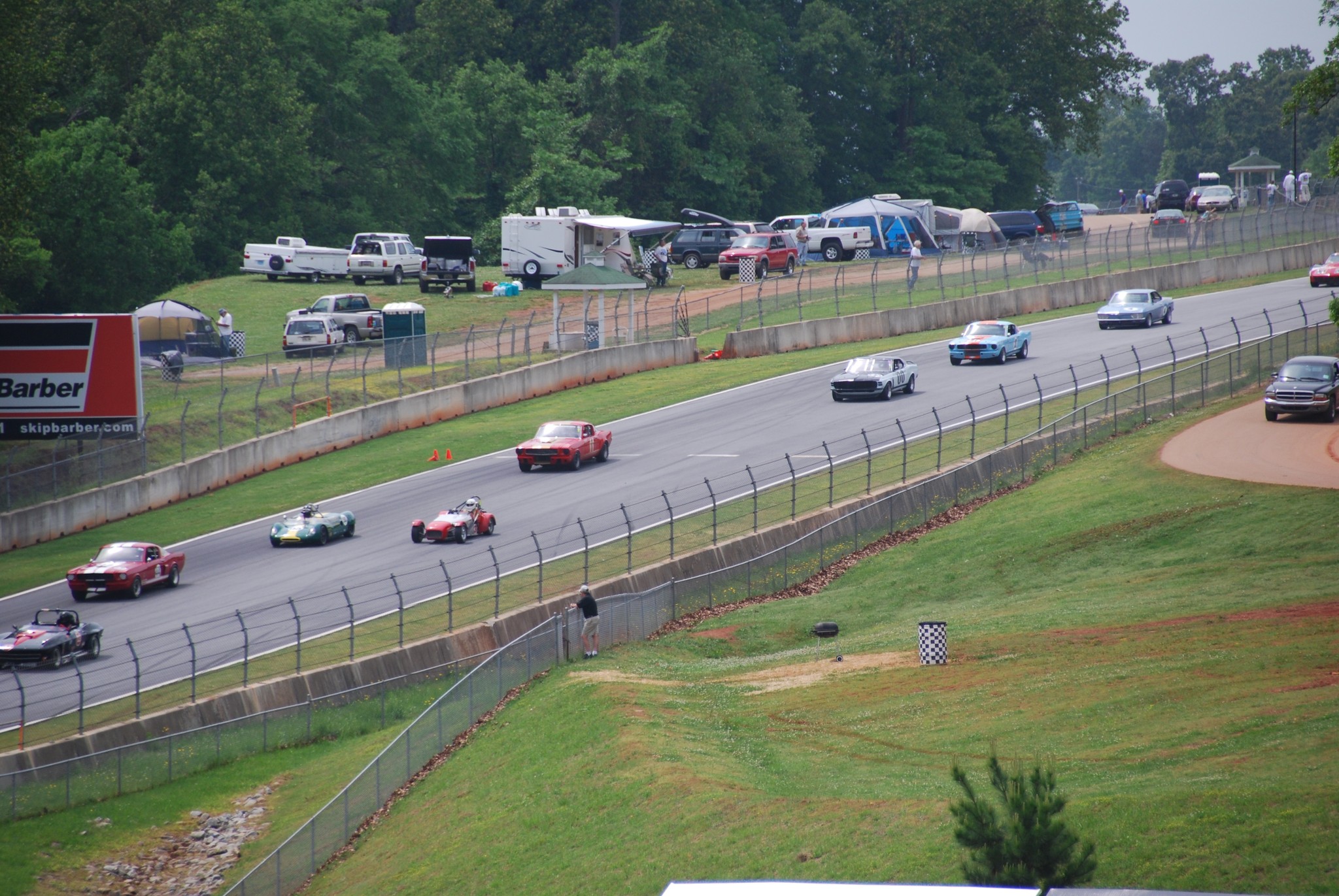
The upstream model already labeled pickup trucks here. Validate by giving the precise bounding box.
[768,215,874,263]
[285,293,383,347]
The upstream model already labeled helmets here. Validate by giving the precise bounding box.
[466,499,476,512]
[59,611,75,627]
[303,505,312,516]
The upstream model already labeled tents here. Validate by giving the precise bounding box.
[132,299,226,357]
[818,195,1009,260]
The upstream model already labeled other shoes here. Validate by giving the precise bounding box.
[582,653,597,659]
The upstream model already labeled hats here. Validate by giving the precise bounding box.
[580,585,589,592]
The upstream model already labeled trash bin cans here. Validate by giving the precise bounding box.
[229,331,245,356]
[918,621,948,664]
[962,232,977,254]
[855,249,869,260]
[161,353,181,381]
[584,321,599,350]
[382,301,428,370]
[739,257,755,283]
[644,248,657,272]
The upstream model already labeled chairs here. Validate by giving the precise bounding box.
[620,259,657,286]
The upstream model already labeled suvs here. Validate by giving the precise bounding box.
[414,235,481,293]
[352,232,424,256]
[718,229,798,280]
[282,314,345,359]
[707,222,775,234]
[346,239,424,286]
[1150,179,1190,215]
[671,207,747,269]
[986,209,1045,252]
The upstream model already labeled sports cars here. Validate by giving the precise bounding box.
[1310,253,1339,287]
[1097,289,1174,330]
[830,356,919,402]
[411,495,497,544]
[66,542,185,601]
[0,609,104,671]
[270,503,356,547]
[515,421,612,472]
[948,320,1031,366]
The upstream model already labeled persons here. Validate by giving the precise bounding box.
[1135,189,1157,214]
[216,308,232,358]
[1021,244,1055,271]
[570,585,599,660]
[906,240,926,293]
[300,505,312,519]
[795,221,809,267]
[654,238,668,288]
[1257,184,1262,206]
[1267,179,1279,210]
[1190,204,1223,250]
[1282,170,1298,205]
[1298,169,1311,204]
[55,611,75,631]
[1118,189,1128,215]
[462,498,481,523]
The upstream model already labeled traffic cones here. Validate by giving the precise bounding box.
[433,449,440,461]
[428,455,437,462]
[446,449,453,460]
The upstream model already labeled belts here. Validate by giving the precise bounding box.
[587,615,598,618]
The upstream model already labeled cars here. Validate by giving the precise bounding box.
[1034,202,1084,239]
[1184,185,1207,212]
[1069,203,1104,216]
[1264,355,1339,423]
[1150,209,1188,238]
[1198,185,1238,214]
